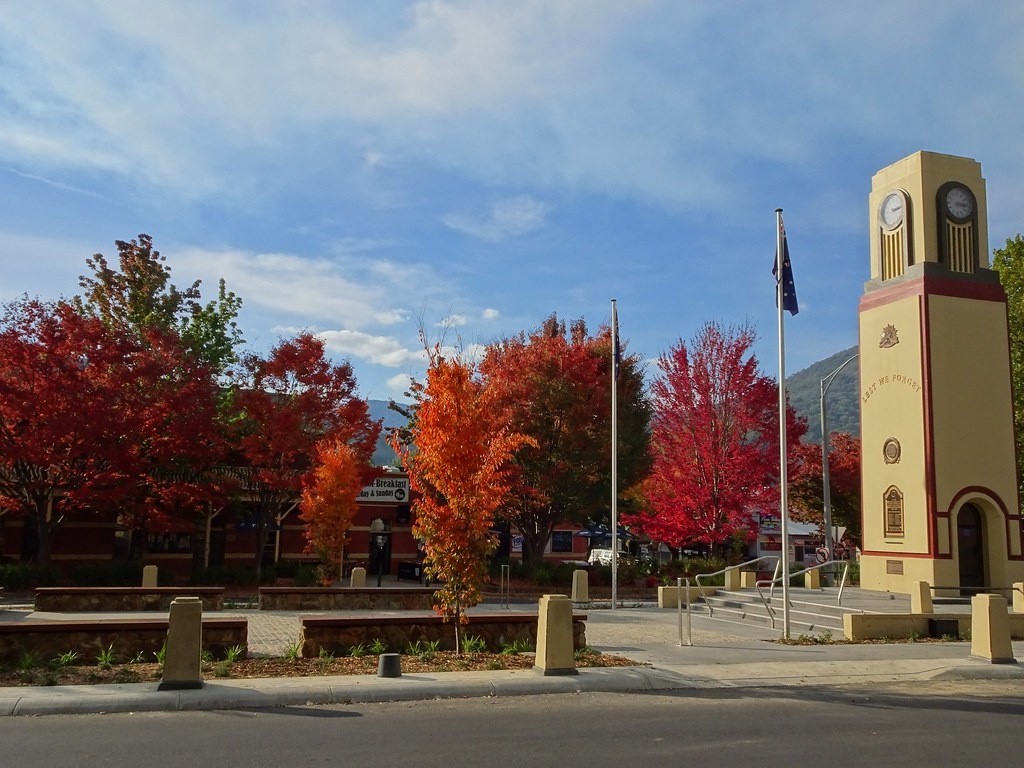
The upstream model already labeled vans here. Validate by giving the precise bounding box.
[589,548,639,566]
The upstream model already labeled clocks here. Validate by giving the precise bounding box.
[877,192,903,231]
[943,183,976,224]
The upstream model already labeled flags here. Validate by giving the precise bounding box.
[612,299,620,383]
[772,213,799,316]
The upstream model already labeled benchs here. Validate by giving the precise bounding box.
[34,587,225,611]
[0,617,248,664]
[299,609,587,658]
[258,587,443,611]
